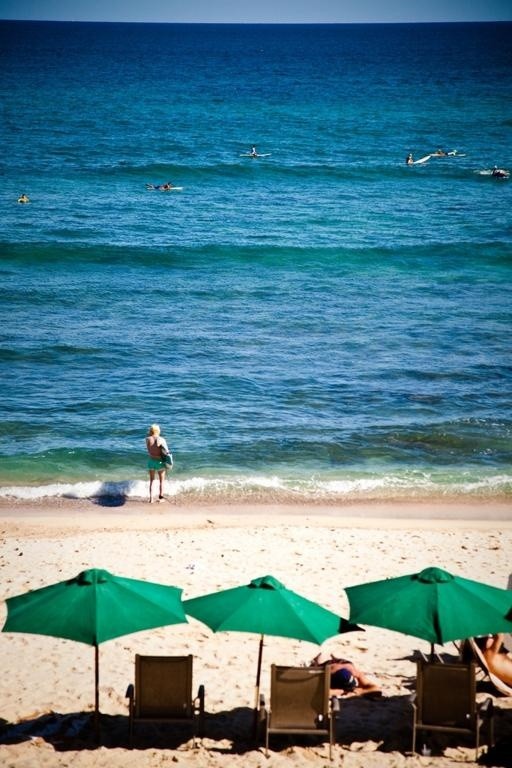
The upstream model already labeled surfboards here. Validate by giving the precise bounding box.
[412,155,432,162]
[239,153,271,158]
[146,186,184,190]
[161,448,173,470]
[429,152,465,158]
[475,169,510,175]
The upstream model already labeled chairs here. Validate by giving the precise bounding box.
[264,663,335,761]
[124,653,206,743]
[411,650,496,762]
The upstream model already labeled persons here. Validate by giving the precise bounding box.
[310,656,379,701]
[473,632,512,689]
[145,183,170,190]
[492,165,508,177]
[145,424,169,504]
[429,149,458,157]
[17,194,30,203]
[251,145,256,157]
[407,153,413,165]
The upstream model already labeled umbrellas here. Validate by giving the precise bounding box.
[181,575,366,713]
[344,567,512,666]
[1,568,188,714]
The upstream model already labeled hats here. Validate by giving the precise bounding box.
[334,668,357,688]
